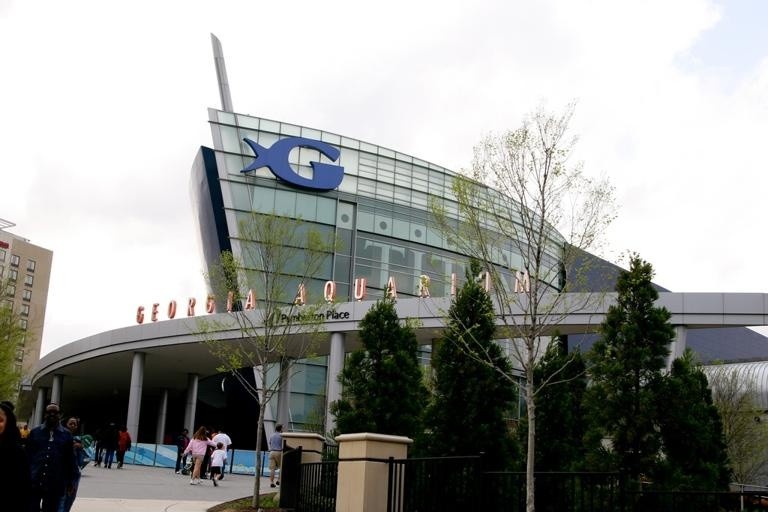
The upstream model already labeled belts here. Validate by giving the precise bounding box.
[270,450,282,451]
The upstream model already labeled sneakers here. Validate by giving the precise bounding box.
[98,463,100,465]
[108,466,111,469]
[276,481,280,485]
[94,463,97,466]
[176,470,224,486]
[271,484,276,488]
[120,465,123,467]
[104,465,107,468]
[117,464,120,468]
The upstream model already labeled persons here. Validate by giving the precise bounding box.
[269,423,285,488]
[1,399,233,512]
